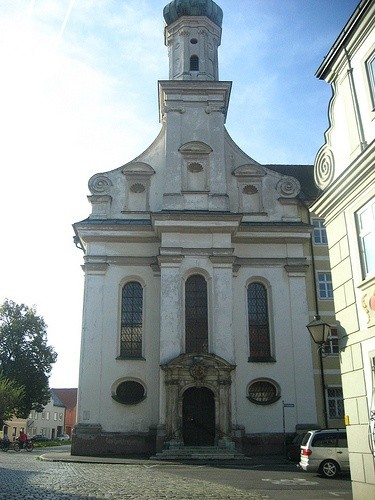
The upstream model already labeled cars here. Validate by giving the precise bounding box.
[29,435,49,442]
[56,434,70,441]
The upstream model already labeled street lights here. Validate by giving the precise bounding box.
[306,314,331,429]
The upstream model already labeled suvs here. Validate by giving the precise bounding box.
[297,427,350,480]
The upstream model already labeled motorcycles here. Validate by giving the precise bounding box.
[11,439,34,452]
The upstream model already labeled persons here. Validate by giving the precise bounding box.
[18,432,27,450]
